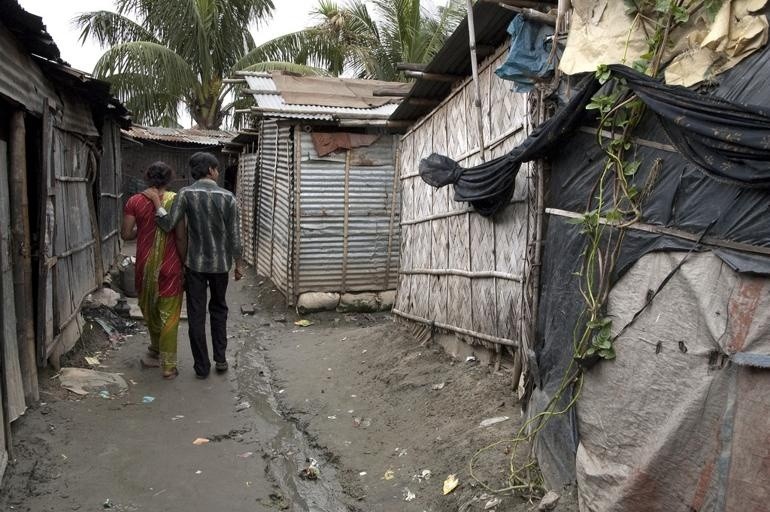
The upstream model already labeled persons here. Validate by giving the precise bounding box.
[121,151,244,380]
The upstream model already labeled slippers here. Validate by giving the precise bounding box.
[147,343,159,358]
[164,367,178,379]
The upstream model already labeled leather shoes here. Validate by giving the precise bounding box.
[194,364,211,379]
[213,360,229,374]
